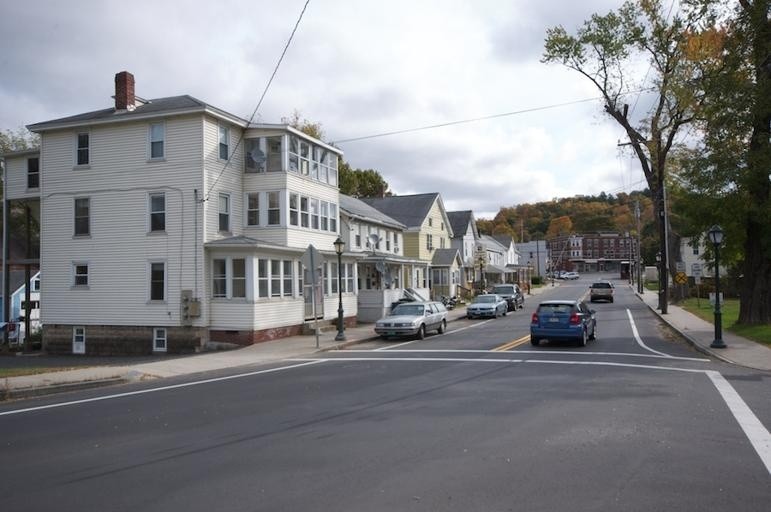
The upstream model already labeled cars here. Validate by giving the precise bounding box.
[375,301,448,339]
[589,280,614,302]
[467,294,507,320]
[546,270,578,280]
[530,300,596,347]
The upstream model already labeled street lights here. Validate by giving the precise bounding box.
[334,238,346,340]
[709,224,727,347]
[656,251,662,309]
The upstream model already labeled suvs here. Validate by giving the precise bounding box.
[488,284,524,311]
